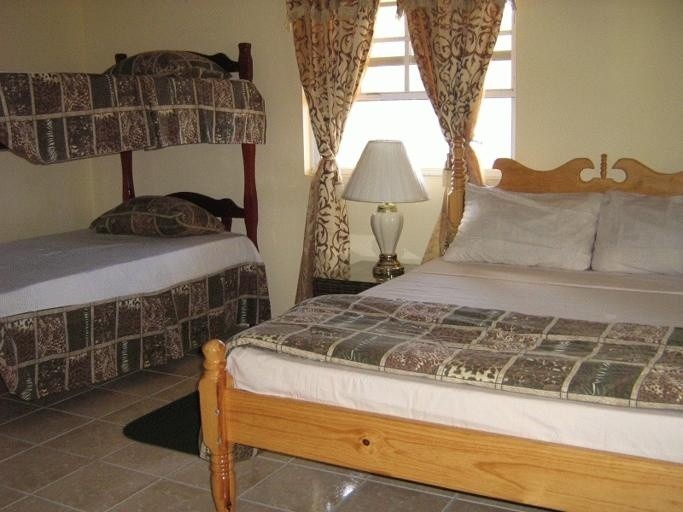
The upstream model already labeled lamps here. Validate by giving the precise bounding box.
[341,140,429,278]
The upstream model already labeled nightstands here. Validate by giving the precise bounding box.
[314,261,420,294]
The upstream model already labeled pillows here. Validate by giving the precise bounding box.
[445,185,602,271]
[89,195,226,236]
[104,50,232,77]
[593,189,683,274]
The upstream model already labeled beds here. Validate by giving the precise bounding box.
[201,153,683,511]
[0,44,273,404]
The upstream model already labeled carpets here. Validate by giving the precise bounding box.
[122,391,200,456]
